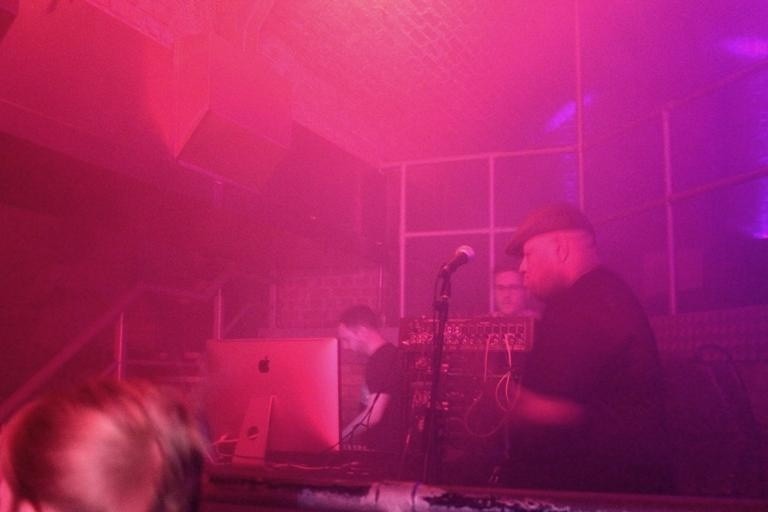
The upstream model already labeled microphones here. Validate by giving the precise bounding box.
[435,244,478,280]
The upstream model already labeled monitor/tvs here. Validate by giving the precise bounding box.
[200,336,347,469]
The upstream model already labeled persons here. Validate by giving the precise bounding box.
[1,377,204,511]
[491,258,528,318]
[338,305,409,469]
[483,201,662,490]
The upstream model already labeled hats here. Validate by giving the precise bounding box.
[502,204,595,259]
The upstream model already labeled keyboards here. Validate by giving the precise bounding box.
[211,466,374,496]
